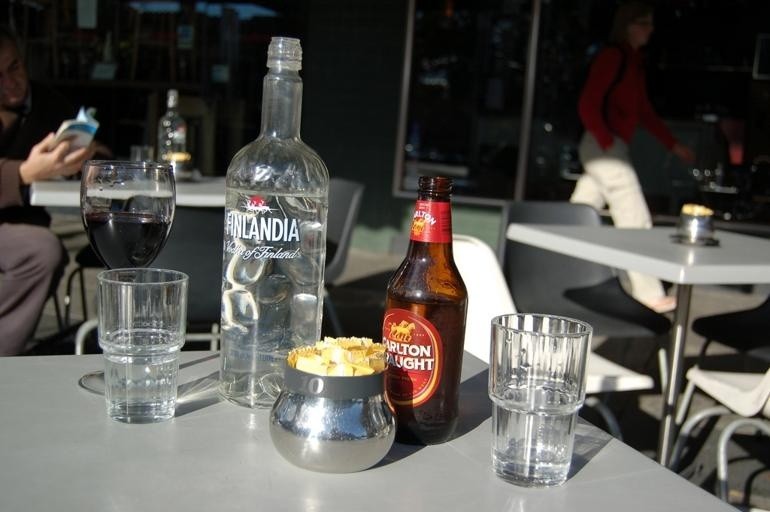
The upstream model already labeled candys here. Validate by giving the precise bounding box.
[294,336,388,376]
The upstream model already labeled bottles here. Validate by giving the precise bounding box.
[157,90,187,166]
[382,176,468,445]
[221,35,330,412]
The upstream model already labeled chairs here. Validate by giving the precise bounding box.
[504,200,671,367]
[142,204,224,322]
[674,365,770,501]
[322,177,365,336]
[452,232,655,443]
[65,243,106,328]
[692,290,770,365]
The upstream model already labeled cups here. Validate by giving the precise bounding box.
[96,267,190,423]
[487,313,592,488]
[131,145,153,181]
[79,159,176,398]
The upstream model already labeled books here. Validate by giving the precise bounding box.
[47,105,101,150]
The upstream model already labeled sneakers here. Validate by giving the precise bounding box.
[647,296,677,315]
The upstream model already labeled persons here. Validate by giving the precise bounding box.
[0,131,95,357]
[0,34,44,131]
[569,9,695,313]
